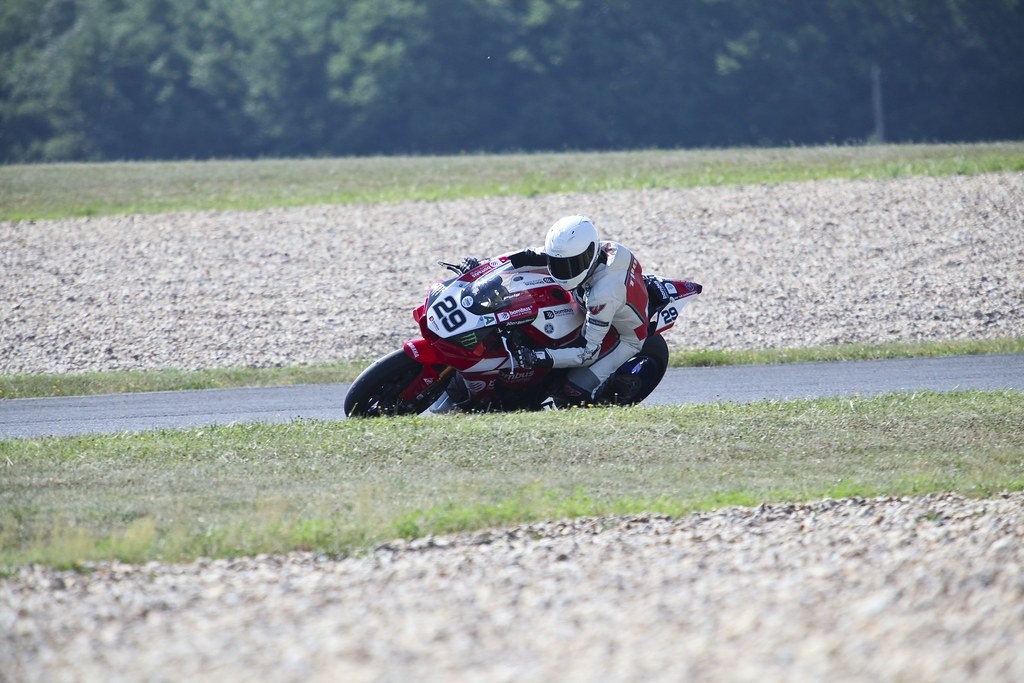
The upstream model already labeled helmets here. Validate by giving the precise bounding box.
[545,216,598,291]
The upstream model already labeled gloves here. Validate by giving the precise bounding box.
[460,256,481,273]
[514,346,553,370]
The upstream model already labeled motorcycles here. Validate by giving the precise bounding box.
[342,251,703,417]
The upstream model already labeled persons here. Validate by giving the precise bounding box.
[459,215,649,412]
